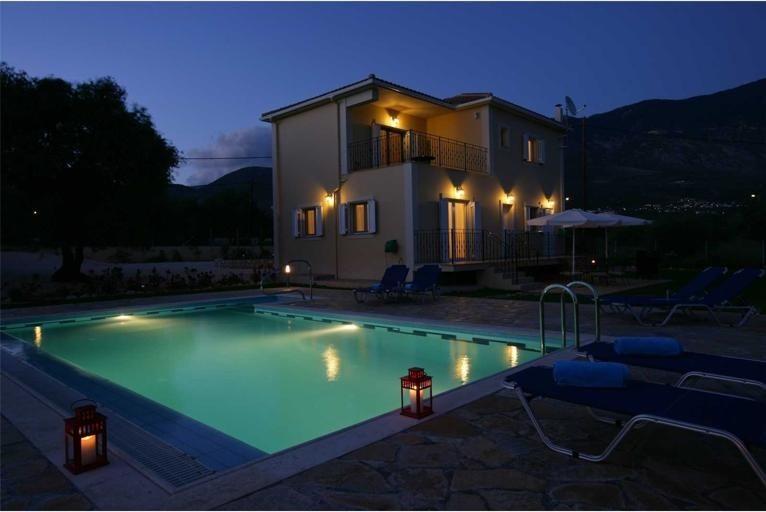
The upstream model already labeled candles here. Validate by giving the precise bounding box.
[80,433,96,464]
[410,386,424,412]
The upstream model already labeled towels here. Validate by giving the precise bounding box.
[613,336,681,357]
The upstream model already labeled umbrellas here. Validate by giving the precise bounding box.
[527,208,621,273]
[599,212,654,259]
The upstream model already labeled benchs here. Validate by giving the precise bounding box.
[500,340,764,489]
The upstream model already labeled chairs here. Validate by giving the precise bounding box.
[588,263,764,327]
[353,264,443,307]
